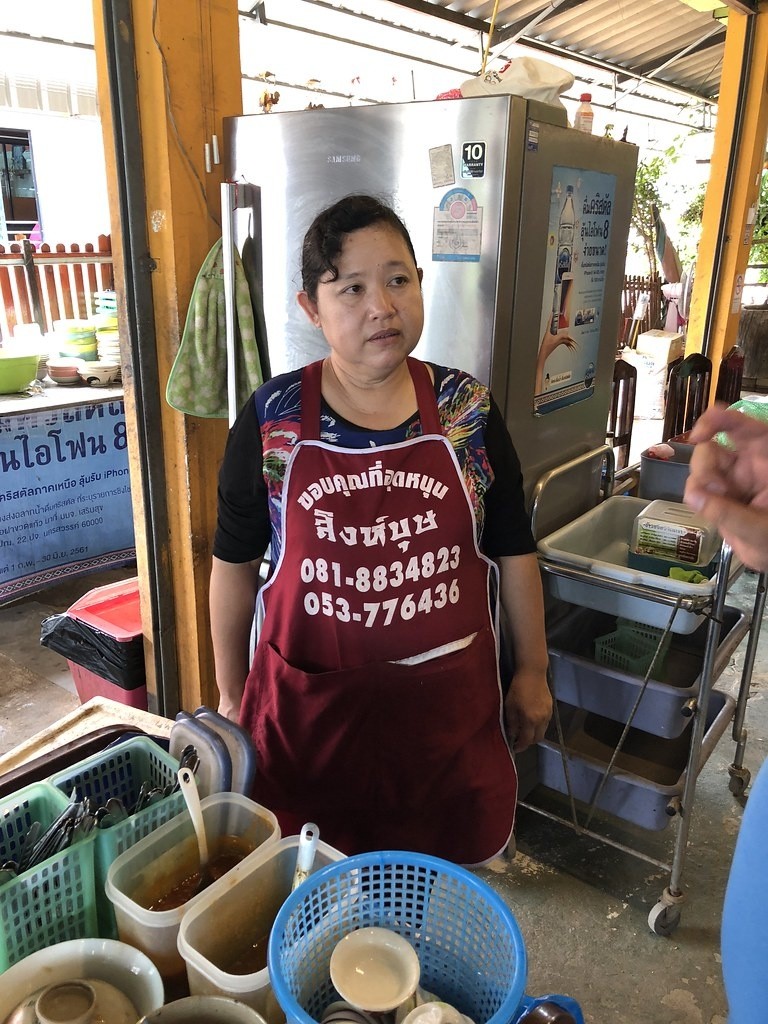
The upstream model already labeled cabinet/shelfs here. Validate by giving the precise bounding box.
[508,444,768,936]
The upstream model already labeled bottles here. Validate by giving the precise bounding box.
[574,94,593,133]
[553,185,576,312]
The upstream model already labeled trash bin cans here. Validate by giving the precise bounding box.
[60,576,149,712]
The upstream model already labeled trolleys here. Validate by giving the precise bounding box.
[501,442,768,937]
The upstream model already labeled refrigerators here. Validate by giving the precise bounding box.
[222,97,637,570]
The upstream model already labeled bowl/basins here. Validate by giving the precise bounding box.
[137,995,267,1024]
[7,978,137,1024]
[0,938,164,1024]
[0,319,119,394]
[322,927,475,1024]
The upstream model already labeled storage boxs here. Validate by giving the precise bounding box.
[547,605,751,740]
[537,496,746,635]
[637,442,695,503]
[538,689,736,833]
[636,329,684,364]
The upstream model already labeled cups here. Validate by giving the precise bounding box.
[558,271,574,328]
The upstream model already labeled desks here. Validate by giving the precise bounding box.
[0,376,136,606]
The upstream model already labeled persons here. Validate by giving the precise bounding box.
[681,406,768,1024]
[202,193,556,927]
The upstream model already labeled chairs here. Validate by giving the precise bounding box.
[714,344,745,406]
[598,359,637,506]
[661,352,713,443]
